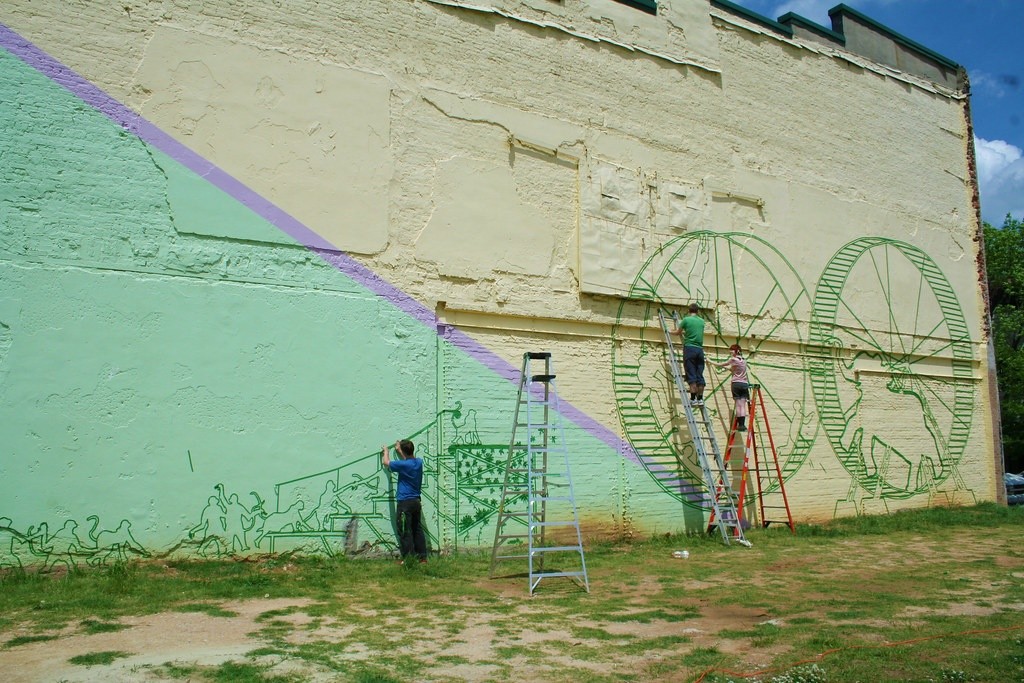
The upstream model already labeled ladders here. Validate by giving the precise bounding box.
[703,385,794,536]
[490,351,589,596]
[656,306,745,546]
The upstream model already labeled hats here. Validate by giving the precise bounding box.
[730,344,742,352]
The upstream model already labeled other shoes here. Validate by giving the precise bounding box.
[690,398,698,406]
[394,560,404,564]
[696,400,704,406]
[417,559,427,564]
[737,426,747,431]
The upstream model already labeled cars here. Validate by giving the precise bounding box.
[1005,470,1024,505]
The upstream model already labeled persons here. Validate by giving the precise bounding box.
[381,439,427,566]
[705,344,750,432]
[667,303,705,406]
